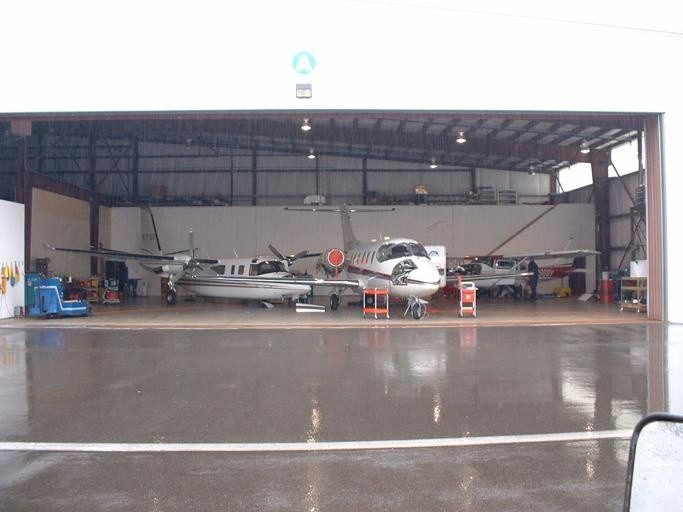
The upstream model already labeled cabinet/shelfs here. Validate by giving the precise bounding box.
[620,277,648,309]
[363,288,389,320]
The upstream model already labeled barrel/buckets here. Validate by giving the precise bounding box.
[600,280,614,302]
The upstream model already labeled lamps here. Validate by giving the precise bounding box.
[308,149,315,159]
[580,138,590,154]
[430,158,437,169]
[302,118,311,131]
[528,165,535,176]
[456,130,466,144]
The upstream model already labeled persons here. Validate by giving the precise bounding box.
[527,257,539,300]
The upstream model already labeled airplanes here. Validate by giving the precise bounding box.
[44,212,326,307]
[268,201,534,320]
